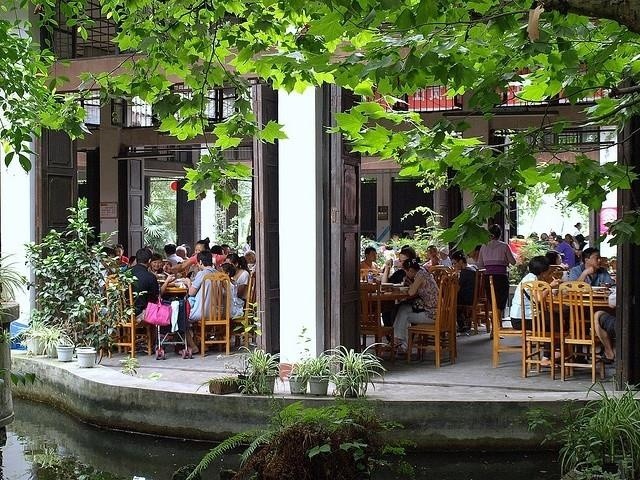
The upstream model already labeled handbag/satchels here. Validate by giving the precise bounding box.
[144,301,172,326]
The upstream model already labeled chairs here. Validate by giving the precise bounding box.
[359,264,619,384]
[86,250,256,361]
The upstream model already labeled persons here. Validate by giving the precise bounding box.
[361,223,617,369]
[93,237,256,354]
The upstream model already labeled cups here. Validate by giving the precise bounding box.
[551,282,616,300]
[157,270,194,287]
[401,282,407,287]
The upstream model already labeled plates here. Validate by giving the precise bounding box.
[393,284,402,287]
[382,283,394,286]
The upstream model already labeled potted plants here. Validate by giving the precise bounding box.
[18,312,98,368]
[561,382,640,480]
[195,342,390,396]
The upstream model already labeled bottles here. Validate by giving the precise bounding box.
[368,271,381,285]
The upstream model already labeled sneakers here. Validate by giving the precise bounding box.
[175,346,208,355]
[599,350,615,364]
[540,349,561,368]
[490,334,504,338]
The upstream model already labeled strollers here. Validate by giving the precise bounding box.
[154,281,194,362]
